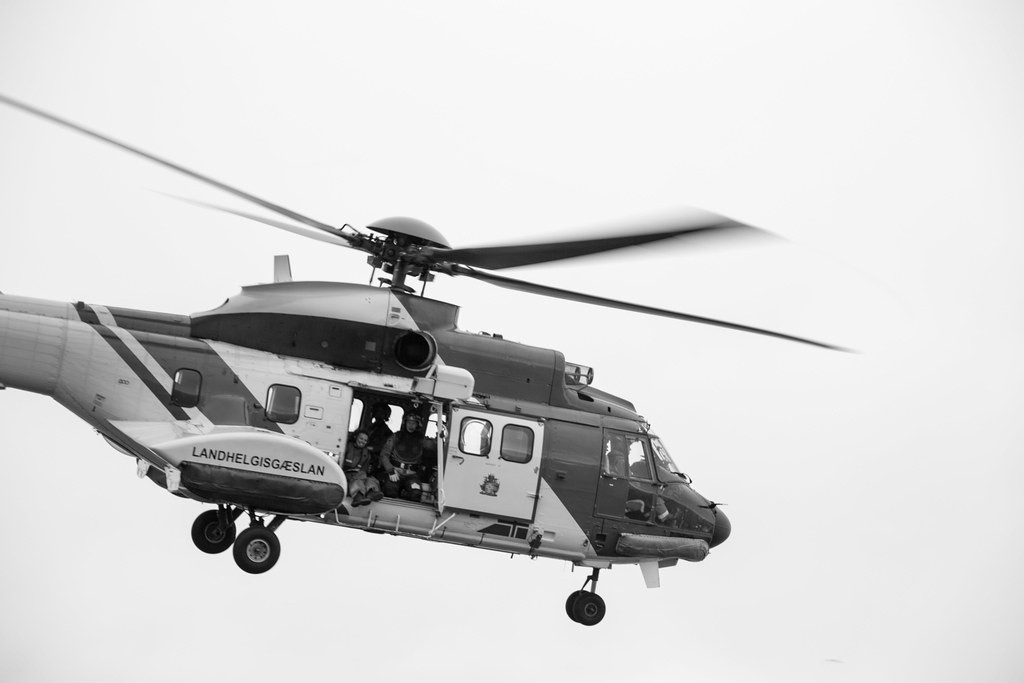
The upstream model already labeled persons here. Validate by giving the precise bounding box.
[607,439,642,488]
[343,431,384,507]
[379,413,427,502]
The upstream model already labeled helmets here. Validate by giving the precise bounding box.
[402,409,420,421]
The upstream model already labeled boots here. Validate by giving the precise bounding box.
[351,492,372,507]
[367,489,384,502]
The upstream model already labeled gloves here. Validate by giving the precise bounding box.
[389,470,400,482]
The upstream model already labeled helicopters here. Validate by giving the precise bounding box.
[0,94,858,626]
